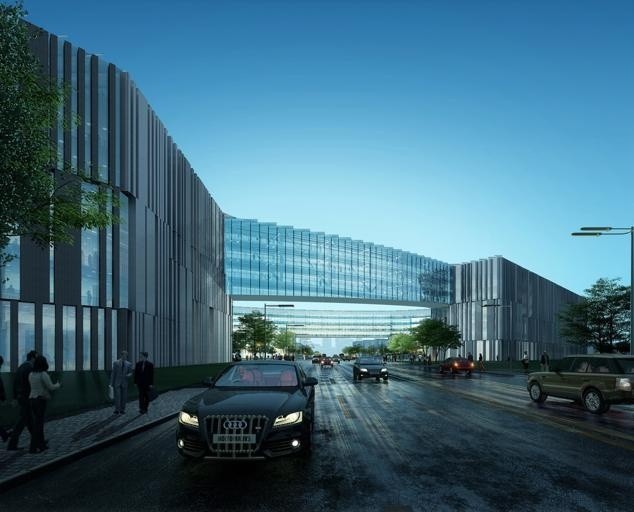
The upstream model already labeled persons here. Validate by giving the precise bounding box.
[540,351,551,372]
[235,349,242,362]
[383,353,397,362]
[133,351,154,414]
[250,353,294,362]
[479,353,486,371]
[409,353,432,365]
[0,350,62,454]
[521,351,529,373]
[109,350,133,414]
[468,351,473,361]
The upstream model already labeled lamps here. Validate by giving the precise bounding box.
[250,368,263,386]
[239,367,255,386]
[276,369,297,386]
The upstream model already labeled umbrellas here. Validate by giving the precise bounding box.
[112,410,147,418]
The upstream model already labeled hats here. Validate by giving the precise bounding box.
[439,357,475,376]
[353,355,389,384]
[176,360,318,467]
[309,354,350,367]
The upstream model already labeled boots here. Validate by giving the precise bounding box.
[527,354,634,414]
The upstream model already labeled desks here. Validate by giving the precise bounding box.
[262,303,295,359]
[482,300,514,361]
[284,324,304,352]
[571,226,634,357]
